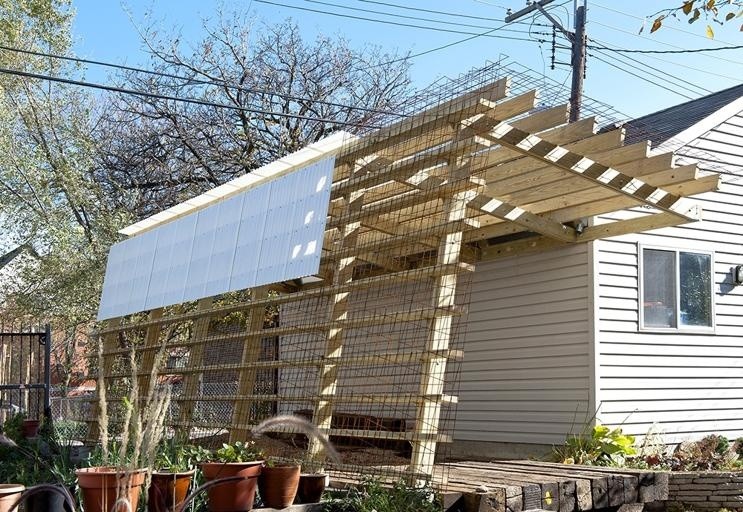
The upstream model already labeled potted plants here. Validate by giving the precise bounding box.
[74,396,275,512]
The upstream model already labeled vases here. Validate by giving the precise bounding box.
[260,461,328,508]
[24,420,40,437]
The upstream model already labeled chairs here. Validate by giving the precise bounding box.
[8,484,132,512]
